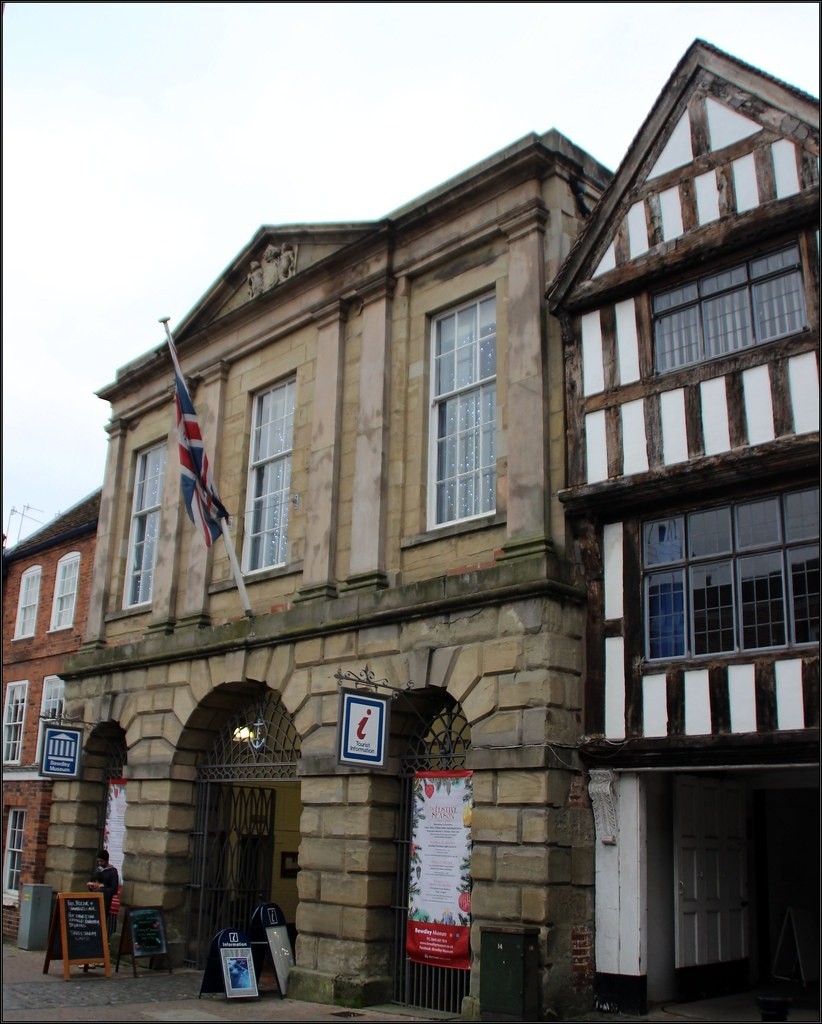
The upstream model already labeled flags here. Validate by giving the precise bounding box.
[172,342,229,546]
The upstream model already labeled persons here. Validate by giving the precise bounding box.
[79,850,119,968]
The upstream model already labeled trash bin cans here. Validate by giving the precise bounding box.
[480,923,544,1019]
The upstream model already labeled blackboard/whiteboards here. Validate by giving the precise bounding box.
[45,892,109,964]
[119,905,170,956]
[249,905,295,995]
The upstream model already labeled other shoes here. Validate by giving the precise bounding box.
[95,963,105,967]
[79,964,96,969]
